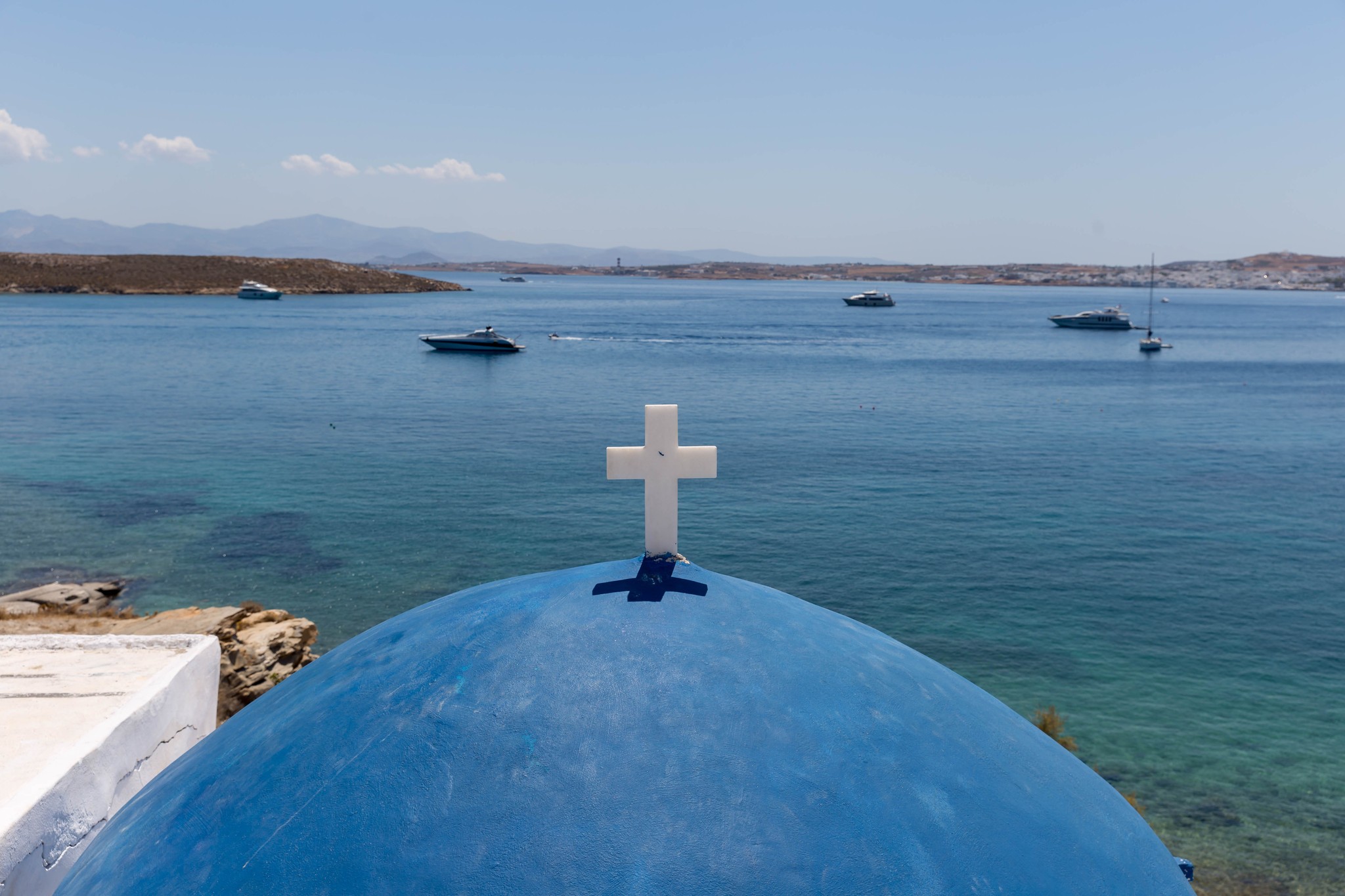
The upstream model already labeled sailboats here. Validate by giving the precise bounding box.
[1139,252,1163,349]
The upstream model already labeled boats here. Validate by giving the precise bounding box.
[843,290,893,306]
[1047,306,1135,328]
[236,280,283,301]
[500,276,525,282]
[417,325,525,352]
